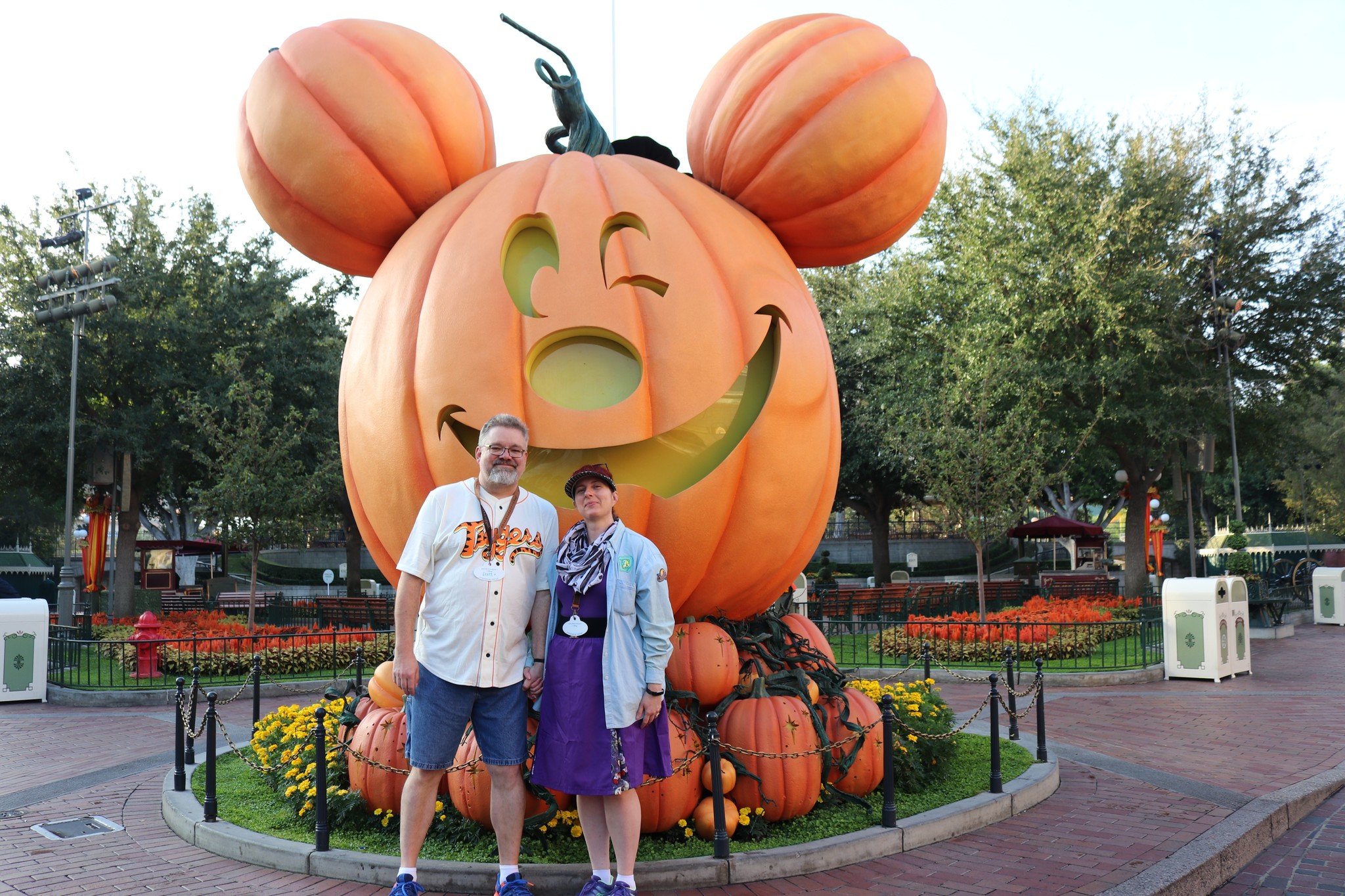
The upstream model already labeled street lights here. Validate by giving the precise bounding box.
[33,179,122,647]
[1209,255,1245,522]
[1115,462,1170,577]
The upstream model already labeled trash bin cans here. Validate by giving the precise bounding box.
[1158,574,1252,684]
[358,579,377,598]
[1,597,49,705]
[1309,566,1345,627]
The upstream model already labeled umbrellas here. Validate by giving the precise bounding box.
[1006,512,1106,570]
[175,534,249,579]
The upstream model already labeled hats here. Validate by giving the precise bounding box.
[564,464,617,499]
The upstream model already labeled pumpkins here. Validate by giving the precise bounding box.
[793,675,820,706]
[368,661,408,708]
[701,757,736,794]
[239,18,496,278]
[692,795,740,841]
[737,671,760,694]
[687,12,948,267]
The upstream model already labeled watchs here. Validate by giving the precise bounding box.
[645,687,665,696]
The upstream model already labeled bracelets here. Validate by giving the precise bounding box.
[533,658,545,664]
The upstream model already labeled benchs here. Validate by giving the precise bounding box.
[312,596,396,631]
[1040,576,1110,598]
[213,592,283,618]
[1248,579,1294,628]
[807,580,1024,635]
[161,595,208,614]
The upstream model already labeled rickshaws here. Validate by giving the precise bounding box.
[1238,556,1327,629]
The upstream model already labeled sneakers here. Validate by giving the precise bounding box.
[575,874,638,896]
[387,873,424,896]
[494,870,534,896]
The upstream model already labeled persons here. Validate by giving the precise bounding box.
[384,413,563,896]
[522,462,680,896]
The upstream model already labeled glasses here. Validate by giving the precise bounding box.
[573,463,610,475]
[481,445,526,458]
